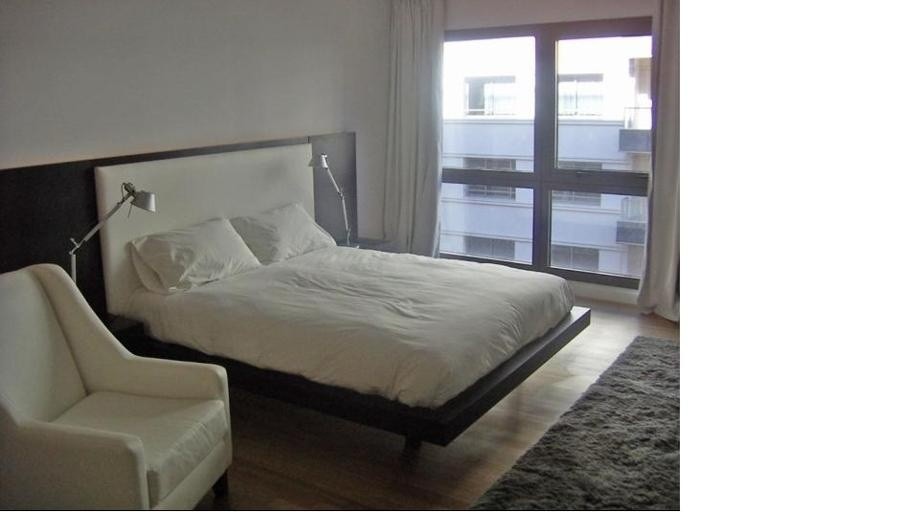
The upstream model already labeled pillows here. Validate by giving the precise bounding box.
[231,199,338,266]
[126,216,262,295]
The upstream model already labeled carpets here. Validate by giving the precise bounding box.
[467,335,679,512]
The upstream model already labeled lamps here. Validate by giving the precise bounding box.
[69,180,157,285]
[307,151,359,248]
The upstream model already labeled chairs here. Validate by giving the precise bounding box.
[0,263,234,510]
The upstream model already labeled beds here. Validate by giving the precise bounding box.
[93,143,592,474]
